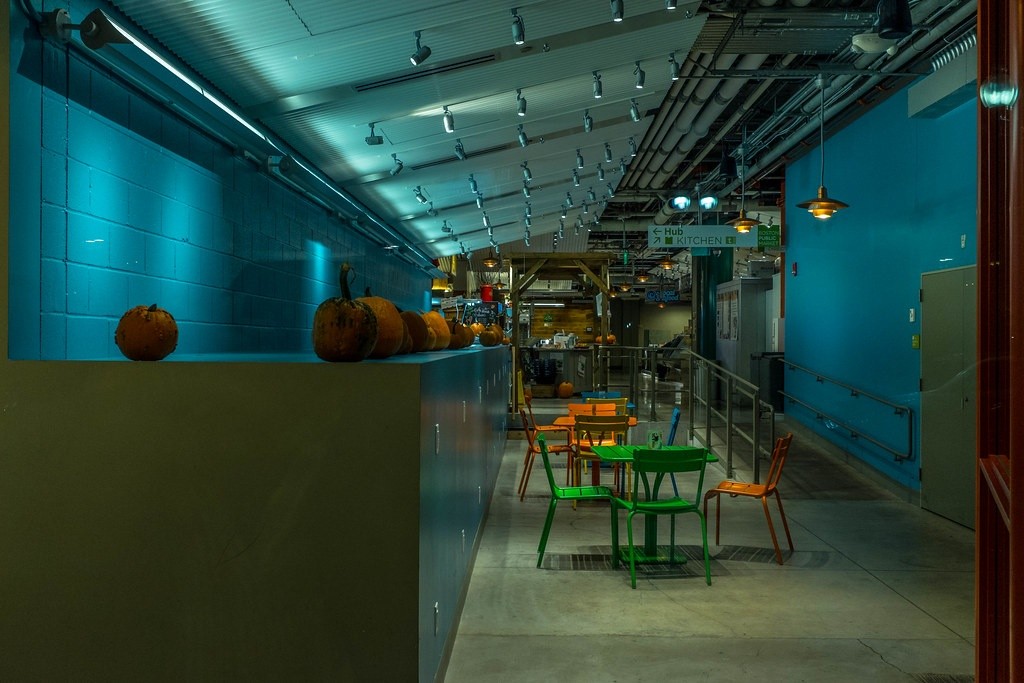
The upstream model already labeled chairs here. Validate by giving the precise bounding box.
[517,387,712,589]
[704,432,794,565]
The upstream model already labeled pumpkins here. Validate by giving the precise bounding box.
[312,265,510,363]
[596,330,616,344]
[559,380,574,398]
[115,303,178,361]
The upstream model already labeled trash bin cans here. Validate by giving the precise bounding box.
[699,358,723,406]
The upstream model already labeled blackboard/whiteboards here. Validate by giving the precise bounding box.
[464,301,499,326]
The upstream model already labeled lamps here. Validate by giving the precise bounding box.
[410,0,677,66]
[767,217,773,226]
[724,126,761,233]
[493,268,507,291]
[798,72,849,219]
[365,53,680,259]
[657,248,679,270]
[50,6,449,280]
[632,235,652,282]
[619,265,632,291]
[482,247,499,268]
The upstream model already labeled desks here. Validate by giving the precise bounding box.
[553,416,637,501]
[590,445,719,565]
